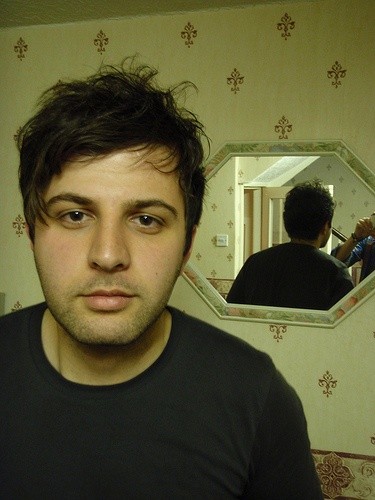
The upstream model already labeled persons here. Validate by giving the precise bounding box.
[225,177,354,311]
[331,212,375,284]
[1,51,327,500]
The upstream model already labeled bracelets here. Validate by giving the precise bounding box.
[351,233,363,242]
